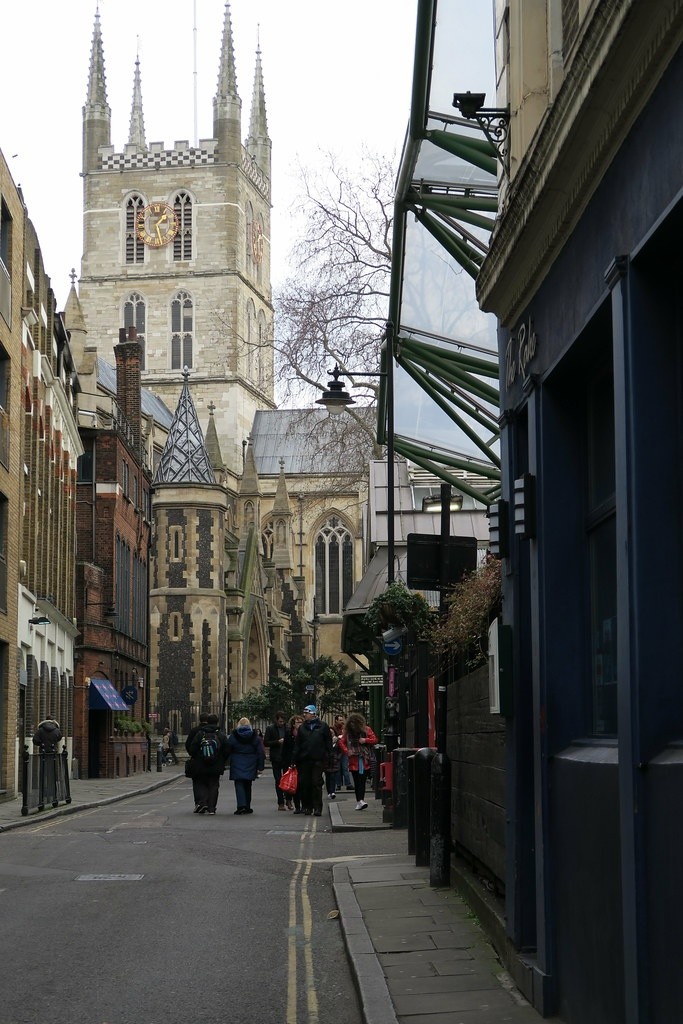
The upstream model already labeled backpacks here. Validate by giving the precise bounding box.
[169,731,179,747]
[198,730,219,762]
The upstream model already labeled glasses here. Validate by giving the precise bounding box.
[303,709,312,713]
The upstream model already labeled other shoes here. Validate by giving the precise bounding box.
[301,807,306,813]
[359,799,368,810]
[346,785,355,790]
[327,794,332,800]
[168,759,173,766]
[305,809,311,815]
[278,803,286,811]
[207,810,216,816]
[355,802,360,810]
[293,808,302,814]
[193,804,203,813]
[331,793,336,799]
[335,785,341,791]
[285,800,294,810]
[198,803,208,813]
[233,807,254,816]
[314,808,321,816]
[162,763,166,767]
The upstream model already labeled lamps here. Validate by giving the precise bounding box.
[488,499,509,562]
[28,617,51,625]
[315,363,356,414]
[512,472,536,541]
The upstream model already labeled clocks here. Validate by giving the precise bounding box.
[135,203,179,247]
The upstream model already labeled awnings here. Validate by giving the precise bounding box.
[341,545,487,655]
[89,679,131,712]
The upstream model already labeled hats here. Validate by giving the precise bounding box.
[303,705,317,716]
[164,728,171,732]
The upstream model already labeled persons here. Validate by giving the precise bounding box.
[295,704,334,816]
[282,715,306,814]
[340,713,378,811]
[229,717,265,815]
[325,715,354,799]
[33,716,63,796]
[185,712,228,815]
[263,710,295,811]
[161,728,179,766]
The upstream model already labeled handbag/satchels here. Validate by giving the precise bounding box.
[369,752,378,778]
[278,767,299,795]
[185,756,194,778]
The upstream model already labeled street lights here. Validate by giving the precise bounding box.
[314,362,395,747]
[421,496,462,888]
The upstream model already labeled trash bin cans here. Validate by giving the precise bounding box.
[380,761,394,809]
